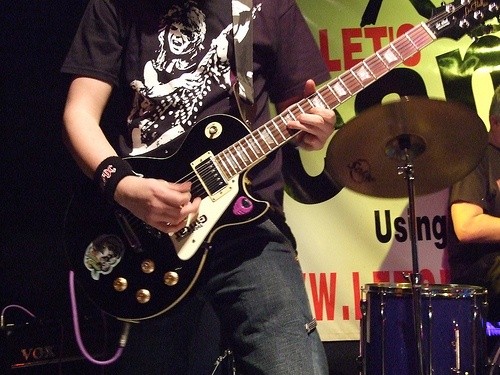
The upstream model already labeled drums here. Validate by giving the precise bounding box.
[364,282,488,375]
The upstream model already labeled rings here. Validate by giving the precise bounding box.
[166,222,172,227]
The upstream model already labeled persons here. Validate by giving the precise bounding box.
[60,0,338,375]
[447,85,500,322]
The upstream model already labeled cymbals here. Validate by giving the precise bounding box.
[326,101,488,198]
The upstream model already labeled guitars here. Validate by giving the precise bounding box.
[65,0,500,323]
